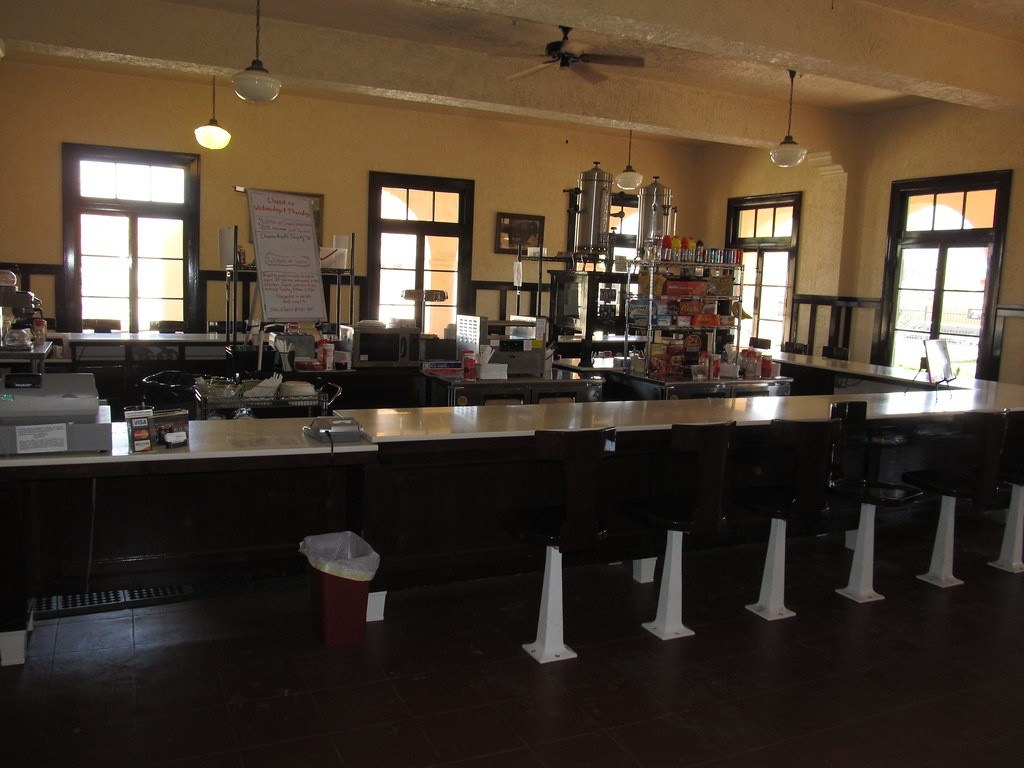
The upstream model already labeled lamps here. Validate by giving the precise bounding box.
[768,70,808,168]
[230,0,282,106]
[194,76,231,150]
[615,130,643,191]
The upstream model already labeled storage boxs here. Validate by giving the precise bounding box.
[634,314,672,326]
[679,299,703,316]
[665,279,707,296]
[676,316,693,327]
[424,368,464,379]
[643,343,667,355]
[692,313,721,326]
[422,361,463,369]
[666,343,685,376]
[476,364,508,380]
[319,246,348,269]
[628,299,668,314]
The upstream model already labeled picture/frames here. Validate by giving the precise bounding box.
[494,212,545,256]
[249,187,325,247]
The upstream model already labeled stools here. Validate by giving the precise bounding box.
[784,342,809,355]
[618,422,737,641]
[986,411,1024,574]
[902,422,1000,590]
[82,319,121,333]
[749,337,771,350]
[829,426,925,604]
[497,427,617,665]
[158,321,184,333]
[11,318,56,330]
[731,418,842,623]
[209,321,247,334]
[821,345,849,362]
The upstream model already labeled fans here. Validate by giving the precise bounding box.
[493,25,645,85]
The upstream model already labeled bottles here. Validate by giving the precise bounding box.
[654,235,704,262]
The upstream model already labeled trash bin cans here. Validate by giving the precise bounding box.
[298,531,380,648]
[123,405,155,411]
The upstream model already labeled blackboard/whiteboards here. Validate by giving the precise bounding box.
[247,188,328,322]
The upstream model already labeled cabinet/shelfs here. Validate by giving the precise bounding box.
[602,370,794,402]
[417,367,606,407]
[623,259,744,379]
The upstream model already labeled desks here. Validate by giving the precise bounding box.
[546,269,639,368]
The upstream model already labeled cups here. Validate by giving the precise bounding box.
[711,354,721,379]
[317,340,327,362]
[463,350,476,382]
[698,352,710,379]
[323,344,334,371]
[742,350,780,380]
[33,319,46,345]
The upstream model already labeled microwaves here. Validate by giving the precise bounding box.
[340,325,420,369]
